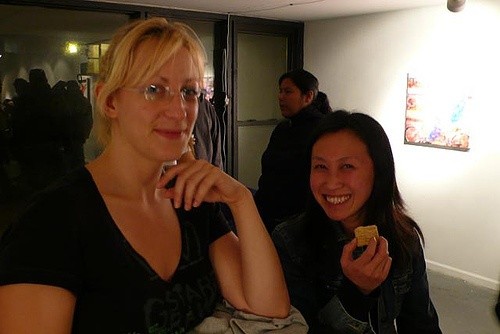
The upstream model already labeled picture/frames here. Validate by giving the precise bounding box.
[404,72,471,153]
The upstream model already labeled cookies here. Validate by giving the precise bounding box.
[354,225,379,248]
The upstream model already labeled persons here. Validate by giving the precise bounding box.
[0,16,291,334]
[192,95,224,171]
[1,69,93,167]
[269,109,442,334]
[251,69,334,230]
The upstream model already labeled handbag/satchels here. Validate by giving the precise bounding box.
[189,301,309,334]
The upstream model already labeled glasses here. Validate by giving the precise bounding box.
[120,83,212,103]
